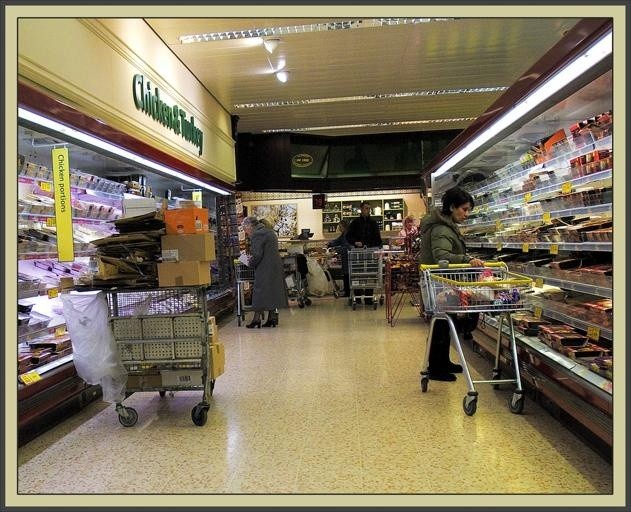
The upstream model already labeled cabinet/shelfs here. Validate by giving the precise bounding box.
[459,134,616,398]
[127,194,246,336]
[321,197,405,235]
[17,166,126,433]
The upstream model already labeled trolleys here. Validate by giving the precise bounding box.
[415,260,536,419]
[281,252,311,310]
[59,284,219,428]
[232,256,280,328]
[346,244,384,310]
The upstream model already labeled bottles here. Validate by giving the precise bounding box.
[333,213,340,222]
[397,213,402,220]
[386,224,391,231]
[333,204,339,211]
[324,215,331,221]
[329,224,332,232]
[332,224,335,232]
[385,202,389,209]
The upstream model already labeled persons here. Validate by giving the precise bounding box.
[326,220,352,297]
[396,216,419,247]
[419,188,484,381]
[346,202,382,305]
[241,216,288,328]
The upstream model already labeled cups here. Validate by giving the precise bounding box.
[323,205,330,212]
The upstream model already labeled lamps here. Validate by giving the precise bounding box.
[262,37,289,83]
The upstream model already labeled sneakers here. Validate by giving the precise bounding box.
[429,362,462,381]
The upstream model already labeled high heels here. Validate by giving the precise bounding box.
[263,318,277,327]
[246,319,261,328]
